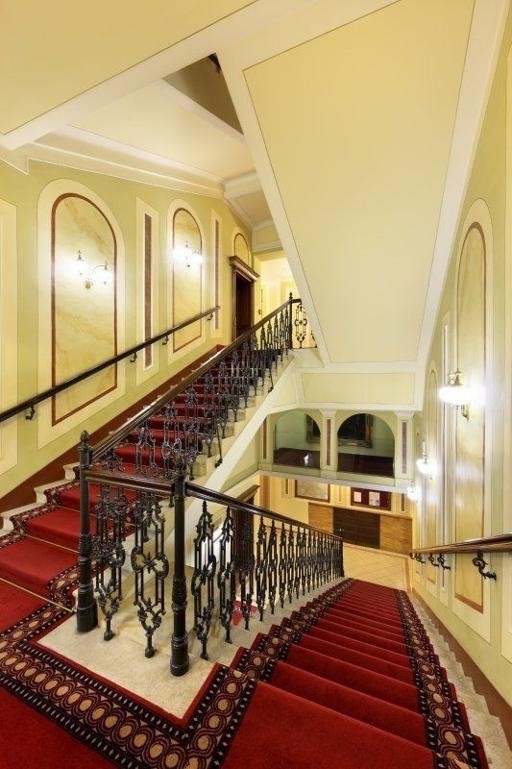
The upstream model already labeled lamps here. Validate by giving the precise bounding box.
[173,243,203,269]
[436,369,482,418]
[416,455,435,481]
[73,251,113,288]
[406,484,421,504]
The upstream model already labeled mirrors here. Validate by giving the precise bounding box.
[336,414,395,479]
[272,411,321,470]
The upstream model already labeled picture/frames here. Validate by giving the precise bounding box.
[306,413,373,448]
